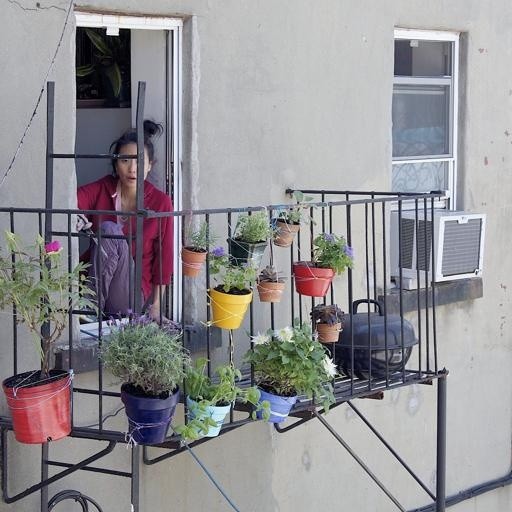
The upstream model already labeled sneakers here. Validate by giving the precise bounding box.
[78,308,99,323]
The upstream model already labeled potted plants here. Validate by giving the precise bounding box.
[256,264,286,303]
[226,211,272,271]
[311,304,346,344]
[182,222,219,277]
[271,192,327,247]
[172,357,272,438]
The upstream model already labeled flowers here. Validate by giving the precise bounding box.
[96,304,194,398]
[0,231,103,378]
[245,322,337,416]
[312,232,356,276]
[207,247,256,292]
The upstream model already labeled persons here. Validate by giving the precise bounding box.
[77,119,175,324]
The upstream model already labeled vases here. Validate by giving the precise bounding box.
[119,383,180,446]
[254,382,298,425]
[293,261,336,297]
[2,369,70,445]
[209,284,253,331]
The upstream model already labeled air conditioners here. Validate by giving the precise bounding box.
[390,208,486,283]
